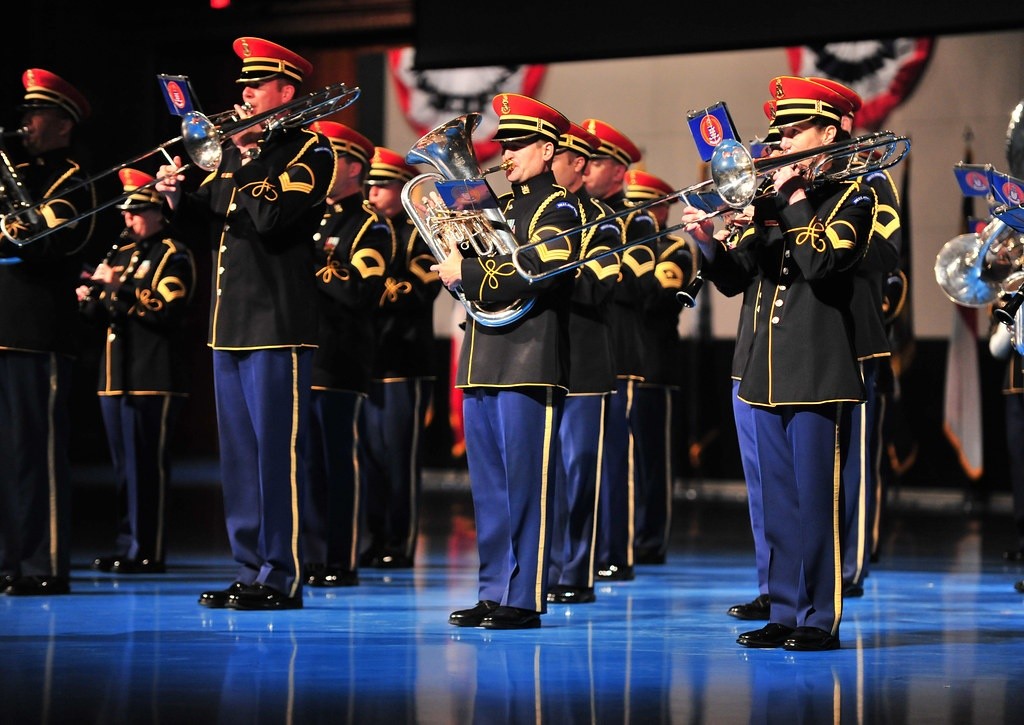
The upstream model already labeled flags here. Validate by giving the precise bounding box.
[944,197,983,477]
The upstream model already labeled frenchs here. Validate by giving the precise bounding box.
[933,215,1024,309]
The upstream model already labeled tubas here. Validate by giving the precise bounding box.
[399,111,539,327]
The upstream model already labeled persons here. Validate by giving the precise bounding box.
[76,168,196,575]
[683,76,907,649]
[156,37,442,610]
[989,290,1024,592]
[430,93,697,629]
[0,69,96,597]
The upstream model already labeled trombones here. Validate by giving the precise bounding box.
[510,129,912,282]
[0,82,362,247]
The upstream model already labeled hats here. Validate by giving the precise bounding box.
[582,119,642,171]
[307,121,375,166]
[232,37,314,85]
[850,145,882,161]
[114,168,166,210]
[769,75,853,128]
[21,68,92,123]
[490,93,570,143]
[558,120,602,158]
[806,76,862,119]
[625,171,678,206]
[764,99,780,133]
[363,147,421,186]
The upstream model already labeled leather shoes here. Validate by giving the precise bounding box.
[3,542,862,658]
